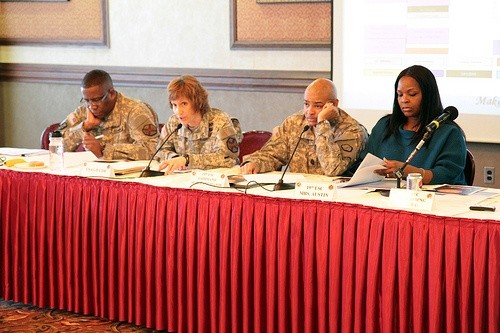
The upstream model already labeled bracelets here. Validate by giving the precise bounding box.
[182,154,190,169]
[419,167,425,181]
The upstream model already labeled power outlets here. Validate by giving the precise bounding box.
[483,166,495,185]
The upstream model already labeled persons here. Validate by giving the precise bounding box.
[359,64,469,189]
[154,74,241,178]
[56,69,159,164]
[240,77,371,178]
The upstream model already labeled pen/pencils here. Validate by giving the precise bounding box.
[95,134,104,140]
[469,206,494,211]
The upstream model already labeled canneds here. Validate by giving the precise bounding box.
[406,173,422,192]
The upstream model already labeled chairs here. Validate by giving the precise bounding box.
[40,122,62,150]
[237,129,274,165]
[464,146,476,186]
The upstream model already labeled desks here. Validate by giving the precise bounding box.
[0,147,500,333]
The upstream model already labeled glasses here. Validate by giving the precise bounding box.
[80,92,110,107]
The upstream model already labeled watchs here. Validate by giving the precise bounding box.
[317,120,330,126]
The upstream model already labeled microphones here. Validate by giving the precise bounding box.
[426,105,459,130]
[273,124,310,190]
[140,124,183,177]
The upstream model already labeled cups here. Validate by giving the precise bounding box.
[48,130,64,171]
